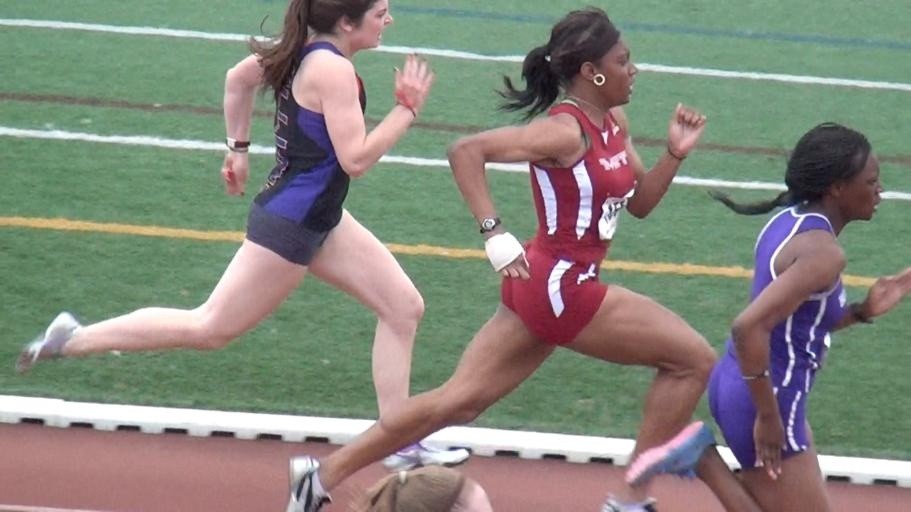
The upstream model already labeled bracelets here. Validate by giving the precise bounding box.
[395,95,417,122]
[666,142,687,164]
[230,147,250,152]
[742,368,773,383]
[226,136,251,148]
[850,300,873,328]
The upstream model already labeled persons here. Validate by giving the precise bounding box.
[365,463,492,509]
[17,2,471,471]
[626,123,911,510]
[284,7,721,509]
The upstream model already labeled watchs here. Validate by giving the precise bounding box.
[479,215,500,235]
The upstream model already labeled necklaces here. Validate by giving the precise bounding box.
[573,91,608,114]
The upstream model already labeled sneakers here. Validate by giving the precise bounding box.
[14,311,79,373]
[625,420,720,488]
[382,440,470,475]
[285,454,334,512]
[602,493,657,512]
